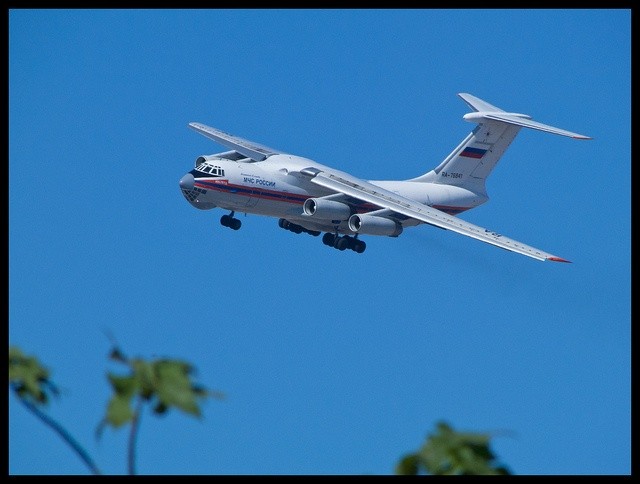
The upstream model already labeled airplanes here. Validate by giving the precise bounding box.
[179,91,595,264]
